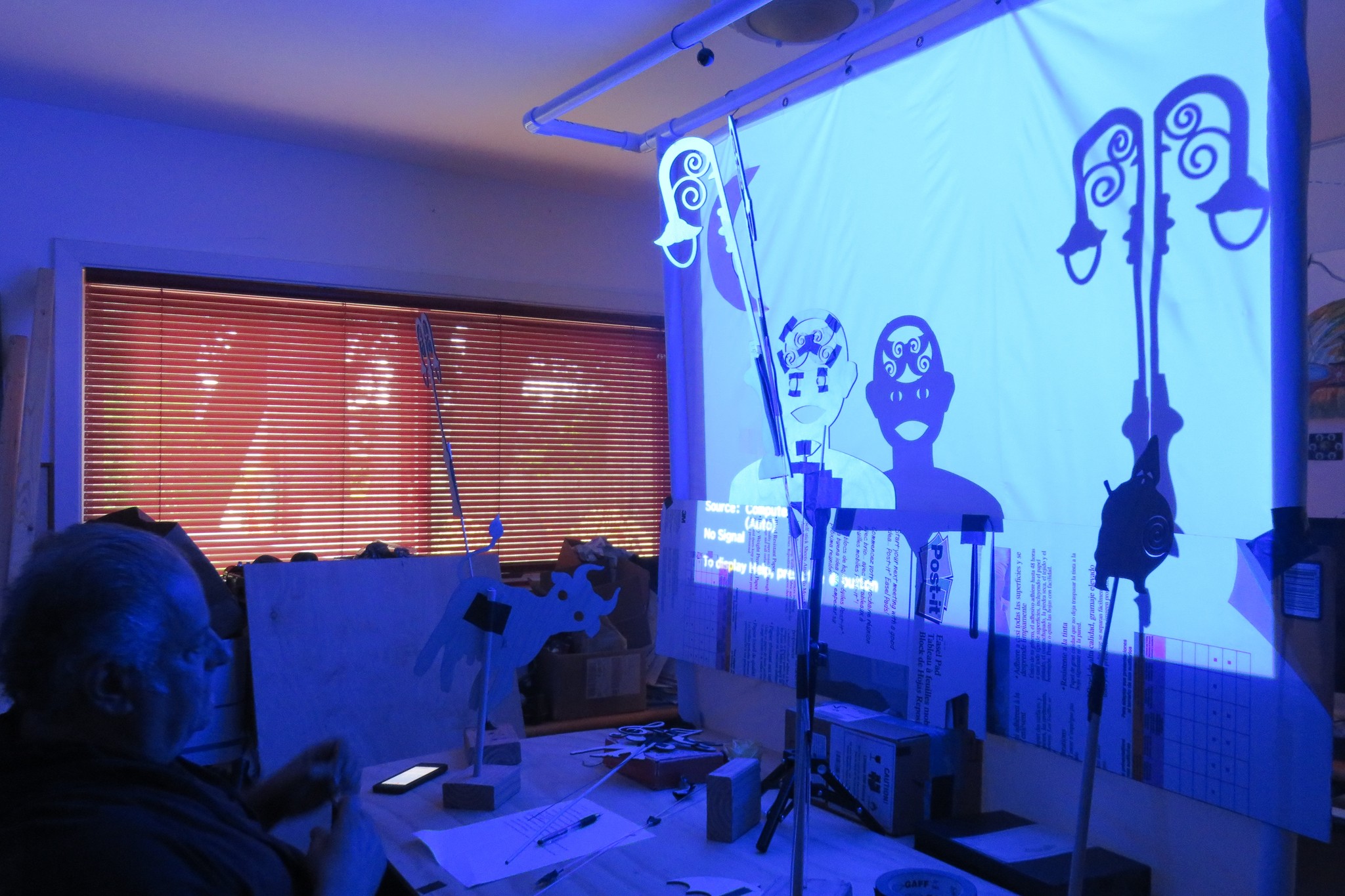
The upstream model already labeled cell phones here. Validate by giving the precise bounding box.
[372,762,449,796]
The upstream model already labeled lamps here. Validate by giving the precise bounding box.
[713,0,875,46]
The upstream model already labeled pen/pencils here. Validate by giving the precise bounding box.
[537,812,604,845]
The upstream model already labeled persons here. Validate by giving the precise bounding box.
[2,519,391,896]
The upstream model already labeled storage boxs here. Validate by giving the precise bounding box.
[913,809,1151,896]
[706,757,761,843]
[784,698,977,838]
[86,507,243,768]
[531,539,649,720]
[603,738,723,789]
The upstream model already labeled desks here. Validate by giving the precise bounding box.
[330,728,1022,896]
